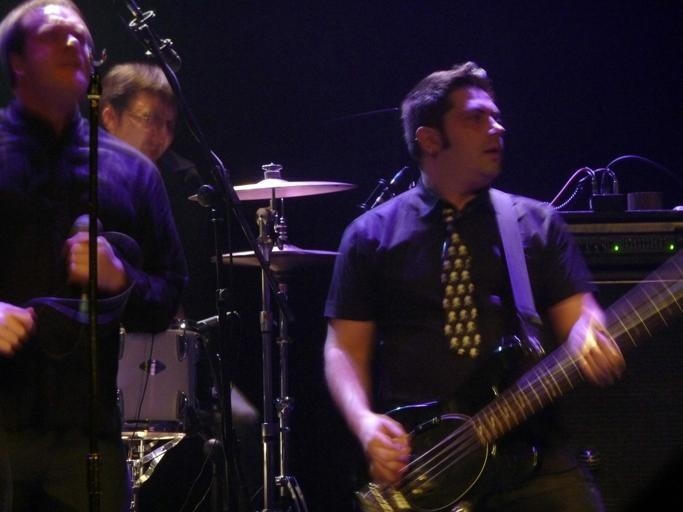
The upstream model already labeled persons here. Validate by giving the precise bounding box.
[1,0,191,511]
[97,64,181,162]
[323,62,626,512]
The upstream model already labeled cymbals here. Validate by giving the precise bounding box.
[212,244,342,272]
[188,179,355,202]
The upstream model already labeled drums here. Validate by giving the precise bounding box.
[115,320,227,442]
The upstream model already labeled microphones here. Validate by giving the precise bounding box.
[126,0,181,72]
[196,310,240,330]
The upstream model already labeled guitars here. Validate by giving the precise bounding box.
[344,249,683,511]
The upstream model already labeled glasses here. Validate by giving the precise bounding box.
[123,111,178,124]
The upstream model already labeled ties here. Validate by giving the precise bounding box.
[439,209,481,358]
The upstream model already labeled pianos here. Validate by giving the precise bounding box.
[556,211,683,233]
